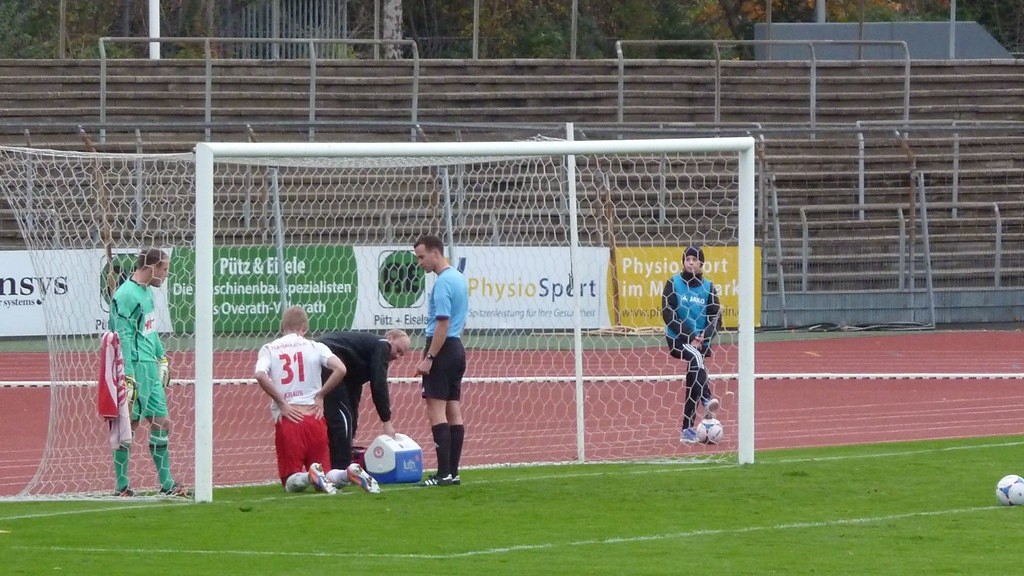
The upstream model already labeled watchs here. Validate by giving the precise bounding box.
[427,353,434,359]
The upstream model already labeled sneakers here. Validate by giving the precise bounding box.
[679,428,701,443]
[115,485,141,497]
[704,398,719,421]
[159,482,195,497]
[412,474,453,487]
[310,463,340,495]
[452,475,461,485]
[347,463,380,493]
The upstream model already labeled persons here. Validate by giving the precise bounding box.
[109,250,194,499]
[413,236,469,486]
[314,329,411,470]
[254,306,381,494]
[661,245,722,443]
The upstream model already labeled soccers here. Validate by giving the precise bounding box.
[995,474,1024,506]
[696,418,723,446]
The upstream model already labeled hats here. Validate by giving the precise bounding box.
[682,244,704,264]
